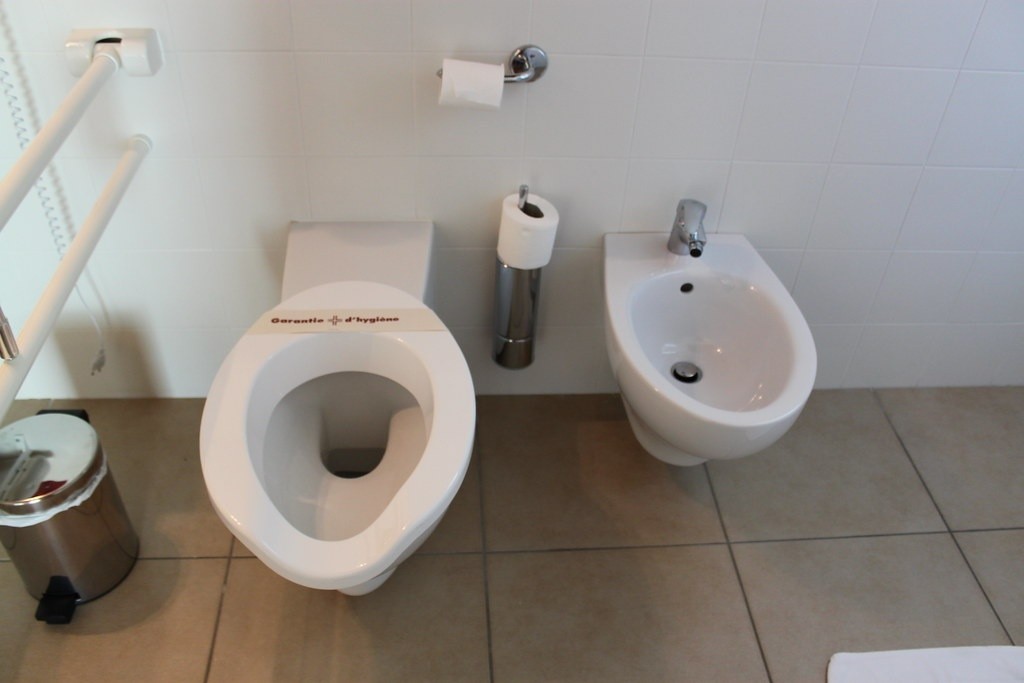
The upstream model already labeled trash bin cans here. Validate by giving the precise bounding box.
[0,406,140,625]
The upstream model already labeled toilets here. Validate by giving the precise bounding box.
[199,219,476,596]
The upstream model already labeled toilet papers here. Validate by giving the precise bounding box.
[497,193,560,270]
[436,57,505,111]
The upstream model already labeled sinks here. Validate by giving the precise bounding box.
[604,231,818,454]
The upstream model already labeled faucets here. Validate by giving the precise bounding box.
[668,198,706,258]
